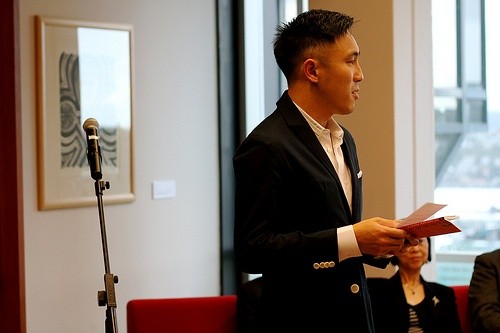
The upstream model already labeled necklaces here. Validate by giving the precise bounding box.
[404,279,420,295]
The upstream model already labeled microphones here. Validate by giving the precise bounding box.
[83,118,103,180]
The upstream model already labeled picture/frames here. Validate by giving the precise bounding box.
[33,14,136,211]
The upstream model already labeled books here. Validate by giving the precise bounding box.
[390,215,462,238]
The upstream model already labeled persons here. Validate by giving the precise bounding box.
[372,236,461,333]
[233,8,419,333]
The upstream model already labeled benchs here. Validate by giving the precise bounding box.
[127,284,470,333]
